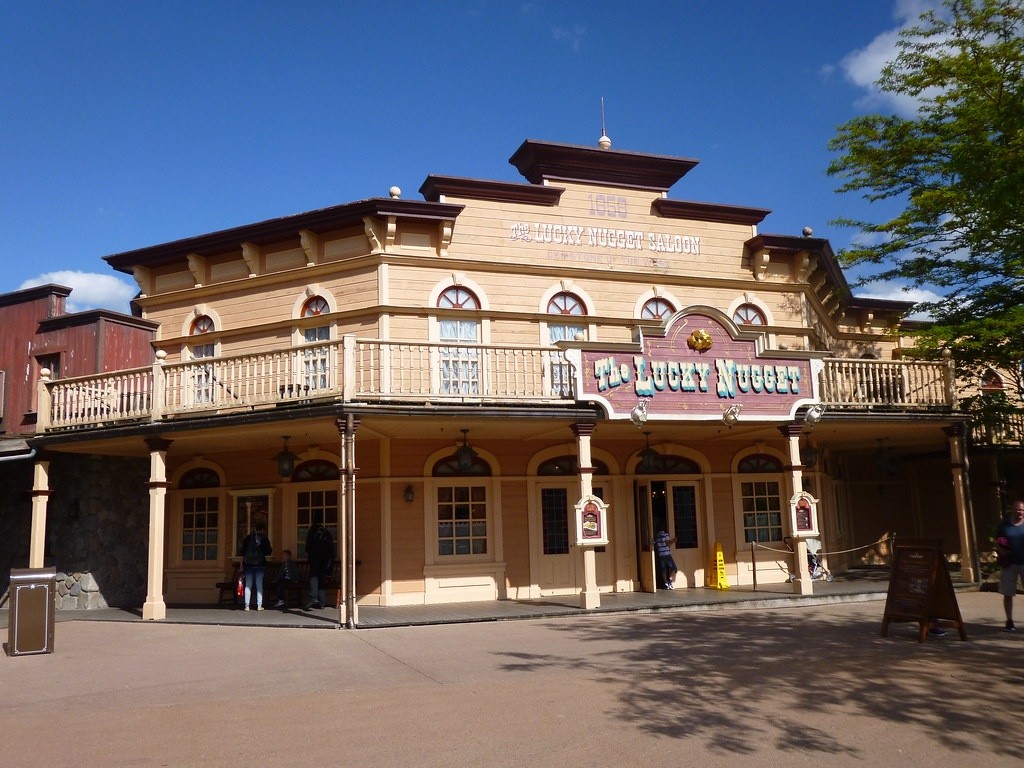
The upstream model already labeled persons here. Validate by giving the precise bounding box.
[992,499,1024,633]
[271,548,299,608]
[928,617,948,635]
[304,512,335,610]
[239,522,274,613]
[655,524,677,589]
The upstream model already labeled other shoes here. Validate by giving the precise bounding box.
[665,579,675,590]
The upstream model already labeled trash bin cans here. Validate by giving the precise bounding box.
[6,566,58,657]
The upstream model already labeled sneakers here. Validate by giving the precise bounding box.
[928,627,948,637]
[1002,619,1016,632]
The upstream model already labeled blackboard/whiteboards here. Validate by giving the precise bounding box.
[884,545,943,622]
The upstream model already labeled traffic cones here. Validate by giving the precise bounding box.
[709,539,730,589]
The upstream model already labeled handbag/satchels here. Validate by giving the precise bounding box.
[238,572,245,597]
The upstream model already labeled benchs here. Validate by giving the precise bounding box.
[216,560,361,609]
